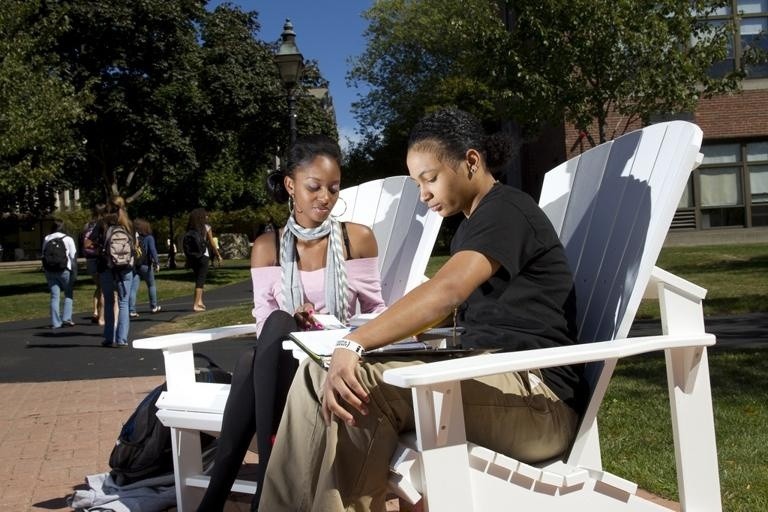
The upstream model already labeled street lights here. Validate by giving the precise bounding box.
[270,16,304,174]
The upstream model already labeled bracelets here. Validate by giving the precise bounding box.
[333,339,366,359]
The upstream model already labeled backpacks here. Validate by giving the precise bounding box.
[102,223,134,271]
[42,238,68,274]
[182,230,207,263]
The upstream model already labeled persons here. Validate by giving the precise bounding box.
[195,133,390,512]
[164,241,177,268]
[41,219,78,329]
[210,232,220,267]
[182,208,223,312]
[84,203,107,326]
[84,195,139,348]
[256,105,590,512]
[129,218,162,318]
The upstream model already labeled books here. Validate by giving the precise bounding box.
[286,327,473,372]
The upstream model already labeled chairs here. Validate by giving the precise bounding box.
[382,119,722,512]
[132,175,446,512]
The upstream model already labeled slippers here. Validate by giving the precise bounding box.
[91,313,100,321]
[130,312,141,318]
[152,305,162,314]
